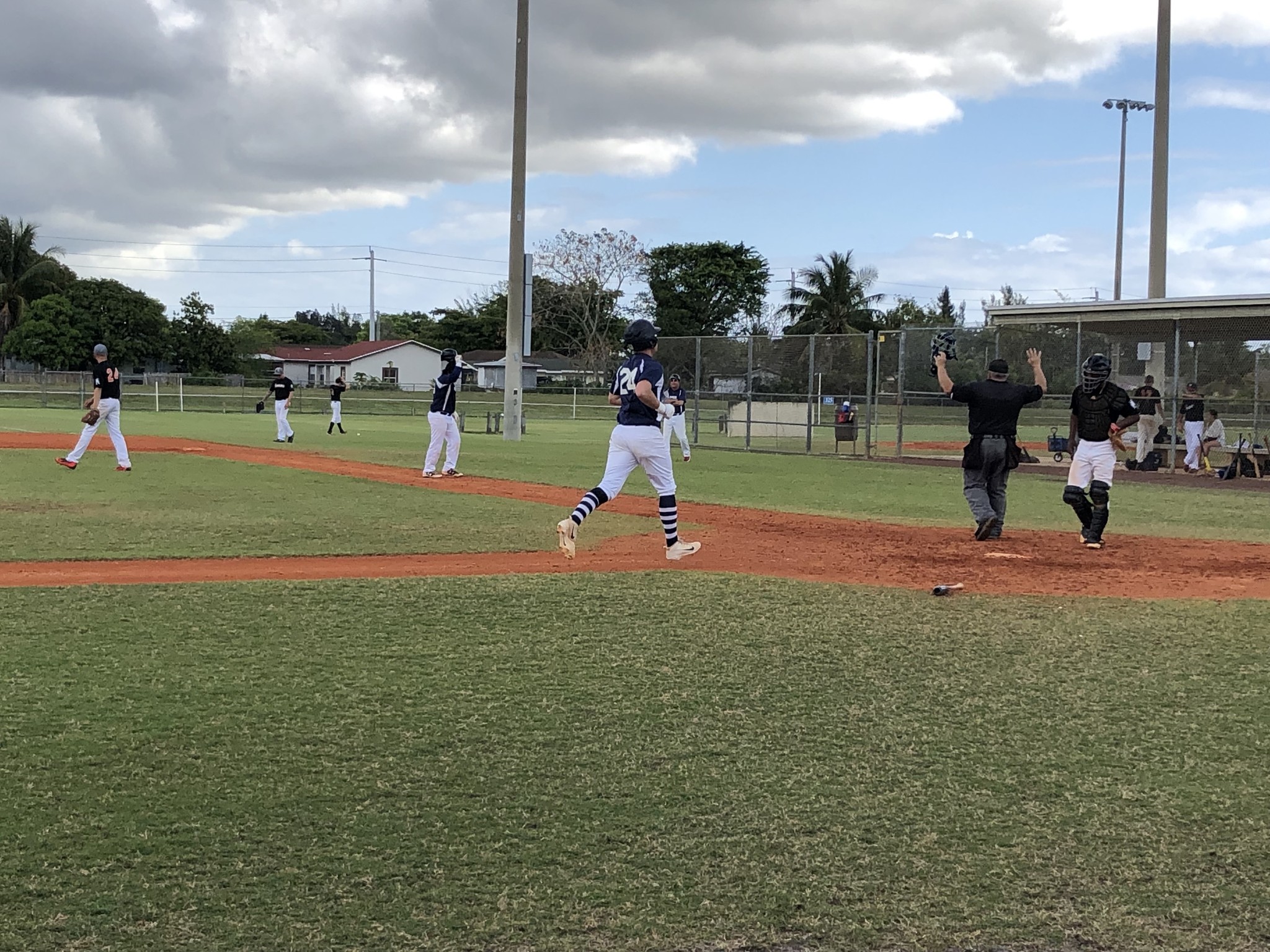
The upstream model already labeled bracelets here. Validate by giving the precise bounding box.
[1110,424,1122,432]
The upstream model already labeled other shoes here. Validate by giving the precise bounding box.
[1079,531,1104,549]
[288,432,294,443]
[975,516,1000,541]
[1184,464,1198,474]
[273,439,285,442]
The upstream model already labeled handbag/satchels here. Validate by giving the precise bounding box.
[1005,436,1022,468]
[962,435,984,468]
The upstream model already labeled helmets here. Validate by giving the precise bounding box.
[1081,353,1112,396]
[930,331,959,374]
[441,348,457,362]
[670,374,682,381]
[623,319,661,349]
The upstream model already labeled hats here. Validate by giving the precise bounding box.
[273,367,285,375]
[336,378,344,385]
[988,359,1010,374]
[1187,382,1198,389]
[94,343,107,352]
[1145,376,1154,384]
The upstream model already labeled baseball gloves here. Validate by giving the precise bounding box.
[81,408,100,426]
[256,401,265,414]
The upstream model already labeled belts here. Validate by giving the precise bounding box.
[441,412,451,416]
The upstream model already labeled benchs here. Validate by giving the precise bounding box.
[1123,442,1270,478]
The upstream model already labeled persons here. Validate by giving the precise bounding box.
[327,376,349,436]
[1184,409,1225,473]
[1181,381,1206,472]
[260,367,295,445]
[1062,352,1139,551]
[1133,374,1167,469]
[54,344,132,471]
[934,347,1047,541]
[422,348,464,479]
[661,373,692,462]
[556,319,702,559]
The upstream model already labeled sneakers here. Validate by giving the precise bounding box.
[114,466,132,471]
[556,515,579,560]
[684,456,691,462]
[663,535,702,560]
[55,458,77,470]
[422,471,443,478]
[442,470,463,477]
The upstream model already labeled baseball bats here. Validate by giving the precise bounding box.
[933,582,965,596]
[1196,433,1260,479]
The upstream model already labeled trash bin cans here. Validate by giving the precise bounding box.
[835,404,858,441]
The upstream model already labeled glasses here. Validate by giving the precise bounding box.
[670,379,677,382]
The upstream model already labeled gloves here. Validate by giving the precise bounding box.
[654,402,675,418]
[455,355,463,368]
[452,411,459,421]
